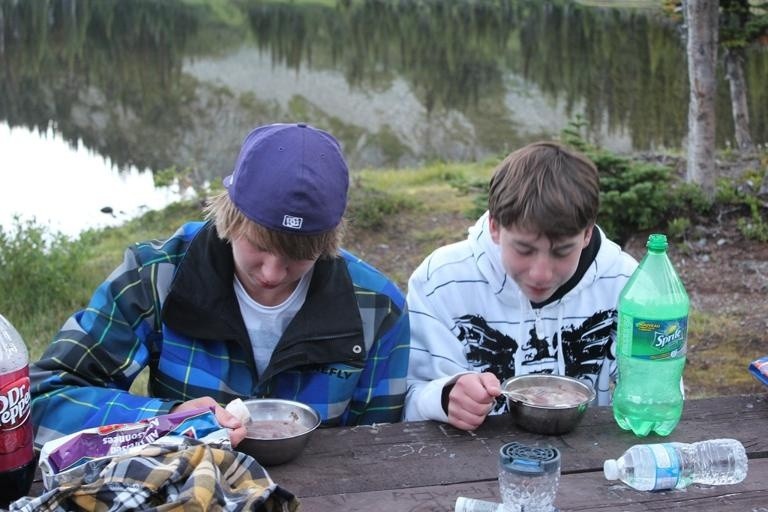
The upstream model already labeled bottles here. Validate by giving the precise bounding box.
[611,232,691,438]
[602,437,751,495]
[0,313,38,511]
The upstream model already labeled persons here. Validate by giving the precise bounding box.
[400,139,690,432]
[27,120,411,460]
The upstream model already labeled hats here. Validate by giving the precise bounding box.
[221,121,351,236]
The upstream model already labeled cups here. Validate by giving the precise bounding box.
[497,441,562,512]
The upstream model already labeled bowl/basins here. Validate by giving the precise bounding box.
[231,397,323,468]
[500,372,598,437]
